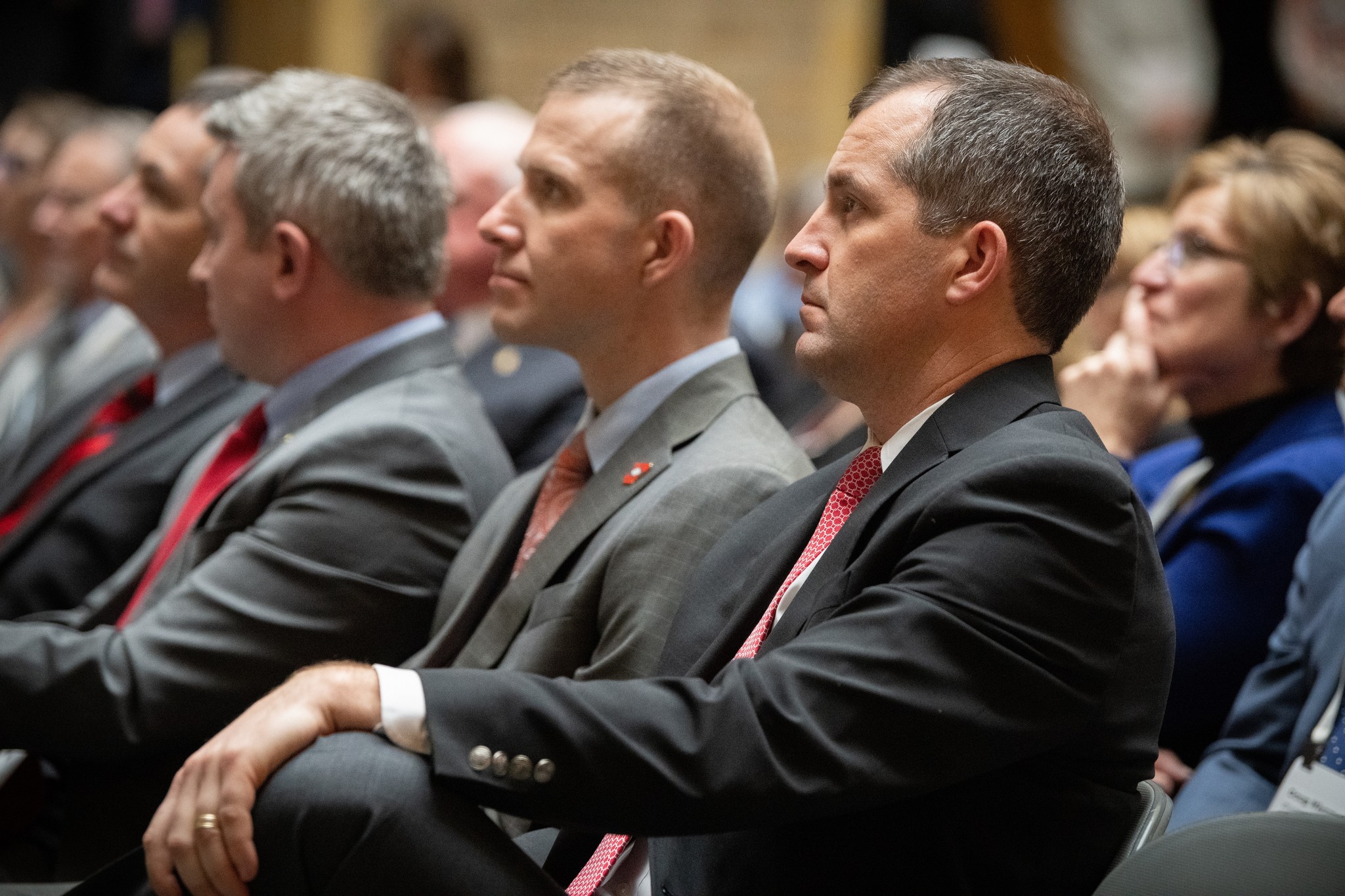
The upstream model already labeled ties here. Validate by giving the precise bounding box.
[510,432,595,586]
[1155,449,1213,536]
[569,442,885,896]
[118,393,271,626]
[0,370,157,538]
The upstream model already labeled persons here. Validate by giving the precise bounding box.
[0,90,96,368]
[0,109,161,466]
[1164,475,1345,835]
[0,68,273,620]
[1059,129,1345,799]
[431,100,588,475]
[0,68,516,896]
[399,49,816,839]
[142,59,1176,896]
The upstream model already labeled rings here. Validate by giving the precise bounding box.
[194,814,217,829]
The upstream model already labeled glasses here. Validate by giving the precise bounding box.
[1146,226,1250,276]
